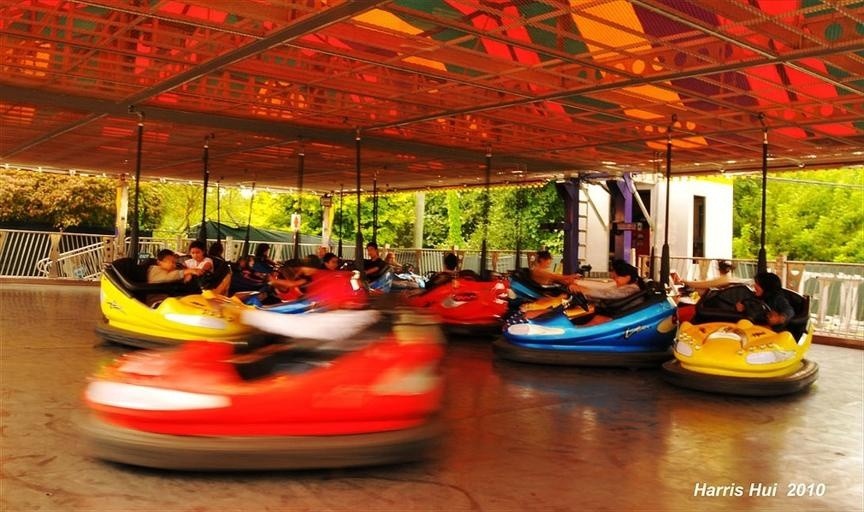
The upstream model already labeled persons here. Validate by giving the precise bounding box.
[569,265,643,328]
[737,271,794,330]
[432,253,459,286]
[680,262,735,296]
[610,260,630,287]
[531,250,576,292]
[150,238,397,311]
